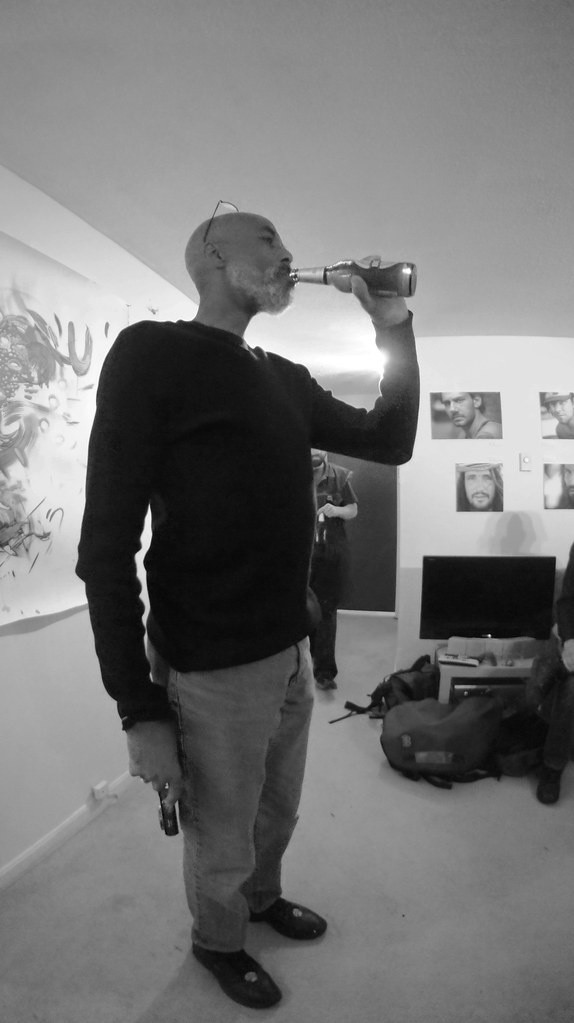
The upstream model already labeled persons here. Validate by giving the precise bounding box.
[310,448,359,690]
[457,462,503,512]
[555,464,574,509]
[74,211,420,1008]
[543,392,574,439]
[537,541,573,805]
[440,392,502,439]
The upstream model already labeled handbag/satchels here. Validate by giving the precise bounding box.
[379,695,504,779]
[367,654,441,717]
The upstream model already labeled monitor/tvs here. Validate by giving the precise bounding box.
[418,557,556,640]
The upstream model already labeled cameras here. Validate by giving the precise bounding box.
[157,786,178,835]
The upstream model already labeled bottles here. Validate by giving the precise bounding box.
[288,259,417,296]
[314,513,327,550]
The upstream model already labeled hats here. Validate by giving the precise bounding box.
[542,392,572,406]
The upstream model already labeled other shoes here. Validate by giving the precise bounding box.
[316,677,337,692]
[536,769,562,803]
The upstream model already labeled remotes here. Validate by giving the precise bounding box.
[439,655,479,667]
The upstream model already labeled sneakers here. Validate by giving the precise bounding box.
[252,896,329,940]
[192,945,283,1009]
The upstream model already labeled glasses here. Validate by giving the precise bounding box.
[203,200,238,244]
